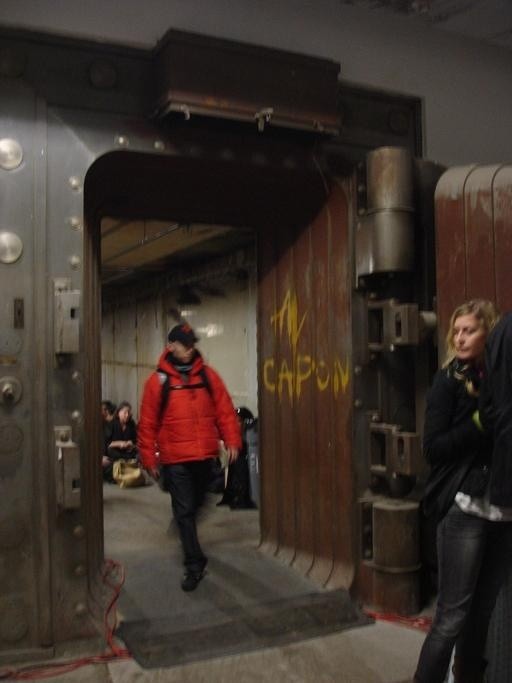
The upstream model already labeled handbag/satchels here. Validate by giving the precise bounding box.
[112,458,146,489]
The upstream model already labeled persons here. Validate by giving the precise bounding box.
[102,401,136,480]
[413,298,512,682]
[135,323,242,591]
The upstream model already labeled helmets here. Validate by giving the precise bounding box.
[235,407,254,419]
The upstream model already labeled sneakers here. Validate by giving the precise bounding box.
[181,557,208,591]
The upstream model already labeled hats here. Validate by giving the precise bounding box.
[168,324,199,347]
